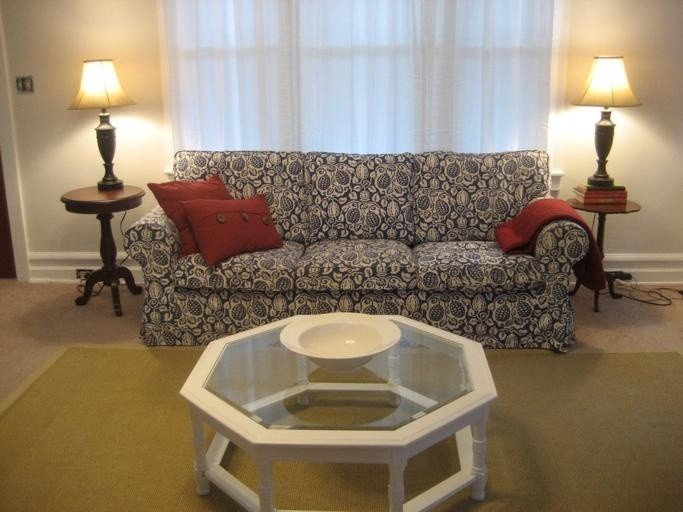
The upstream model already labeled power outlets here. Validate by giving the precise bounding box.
[77,270,92,279]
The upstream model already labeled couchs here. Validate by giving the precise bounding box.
[123,150,590,353]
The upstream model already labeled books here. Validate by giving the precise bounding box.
[572,186,627,206]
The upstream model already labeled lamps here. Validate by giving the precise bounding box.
[570,57,641,186]
[66,60,136,190]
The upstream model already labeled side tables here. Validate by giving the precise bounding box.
[565,198,641,312]
[61,186,145,317]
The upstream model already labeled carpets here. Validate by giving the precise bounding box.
[0,341,683,512]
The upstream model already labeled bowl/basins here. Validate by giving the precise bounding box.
[279,311,398,372]
[283,363,403,430]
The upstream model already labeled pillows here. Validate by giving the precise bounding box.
[147,175,234,258]
[180,195,283,264]
